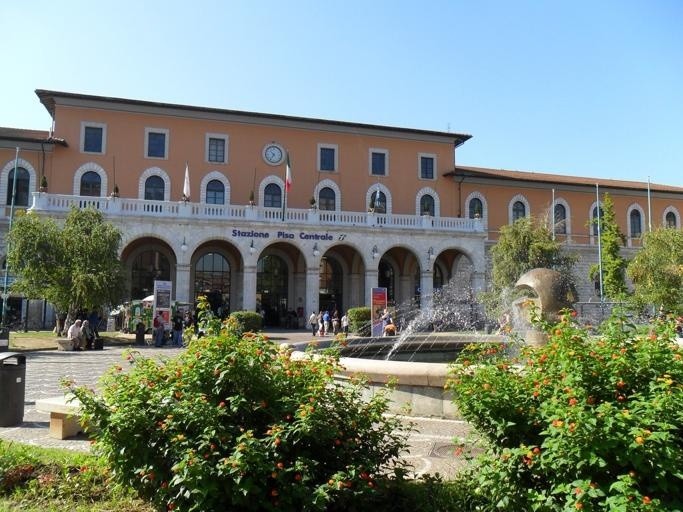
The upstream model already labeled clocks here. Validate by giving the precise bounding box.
[261,143,286,166]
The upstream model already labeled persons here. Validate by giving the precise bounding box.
[309,310,350,336]
[184,311,193,328]
[68,305,101,351]
[152,311,164,347]
[171,310,183,347]
[382,308,393,336]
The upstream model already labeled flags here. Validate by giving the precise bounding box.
[376,186,381,208]
[183,166,191,198]
[286,152,293,192]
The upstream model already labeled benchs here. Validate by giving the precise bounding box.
[56,336,78,351]
[34,392,118,440]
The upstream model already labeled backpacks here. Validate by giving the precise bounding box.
[153,316,161,327]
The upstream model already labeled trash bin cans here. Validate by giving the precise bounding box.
[0,327,9,352]
[0,352,26,426]
